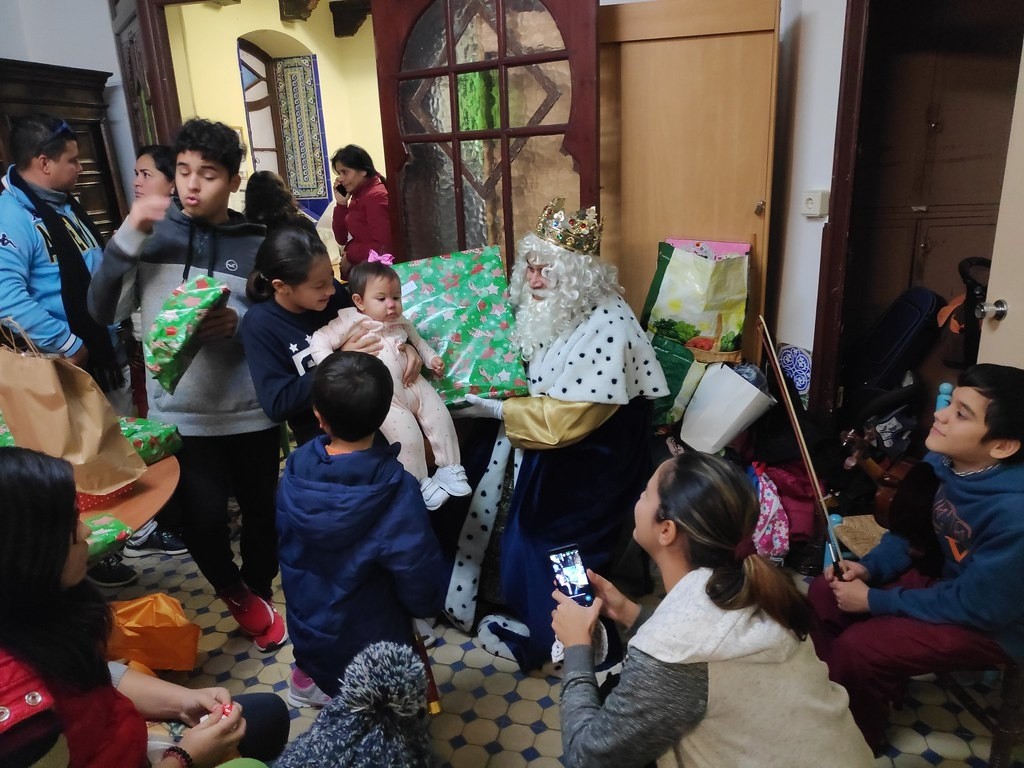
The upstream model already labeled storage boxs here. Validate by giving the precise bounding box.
[116,417,185,465]
[389,243,529,412]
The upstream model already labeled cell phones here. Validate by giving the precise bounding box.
[337,183,347,197]
[547,542,596,608]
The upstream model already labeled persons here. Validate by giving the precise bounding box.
[804,362,1024,753]
[272,352,446,711]
[308,256,472,510]
[550,452,878,768]
[0,444,289,768]
[0,112,423,652]
[440,200,674,672]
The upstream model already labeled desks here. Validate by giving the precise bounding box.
[83,453,181,565]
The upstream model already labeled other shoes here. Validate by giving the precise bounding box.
[846,695,891,754]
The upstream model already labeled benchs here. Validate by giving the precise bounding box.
[826,514,1024,768]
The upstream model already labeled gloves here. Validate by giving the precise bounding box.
[449,393,504,420]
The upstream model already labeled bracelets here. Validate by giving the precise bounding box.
[161,746,192,768]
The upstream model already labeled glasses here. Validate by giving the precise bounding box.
[33,117,73,157]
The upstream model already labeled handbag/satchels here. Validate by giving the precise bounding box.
[0,316,148,513]
[643,241,748,352]
[640,330,743,423]
[681,361,777,454]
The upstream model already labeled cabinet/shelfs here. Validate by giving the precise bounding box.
[0,57,129,362]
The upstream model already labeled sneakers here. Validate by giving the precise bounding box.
[252,597,289,653]
[123,529,190,556]
[86,556,138,587]
[416,476,449,510]
[431,463,473,496]
[220,577,274,636]
